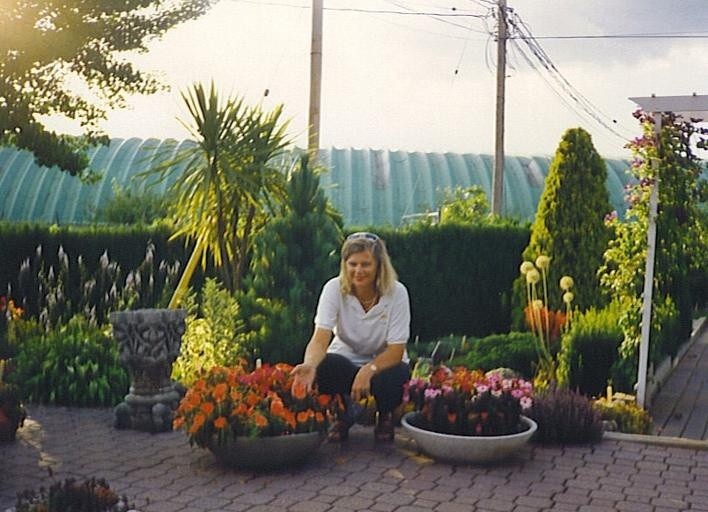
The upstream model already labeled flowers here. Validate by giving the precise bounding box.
[402,368,533,436]
[172,358,344,450]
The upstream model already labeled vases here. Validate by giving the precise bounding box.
[401,412,537,463]
[206,431,322,472]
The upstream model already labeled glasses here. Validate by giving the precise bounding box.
[346,231,379,243]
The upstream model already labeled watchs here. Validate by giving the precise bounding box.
[371,363,376,371]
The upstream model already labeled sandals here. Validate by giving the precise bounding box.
[327,417,354,443]
[374,413,395,442]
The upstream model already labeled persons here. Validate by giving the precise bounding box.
[290,231,412,443]
